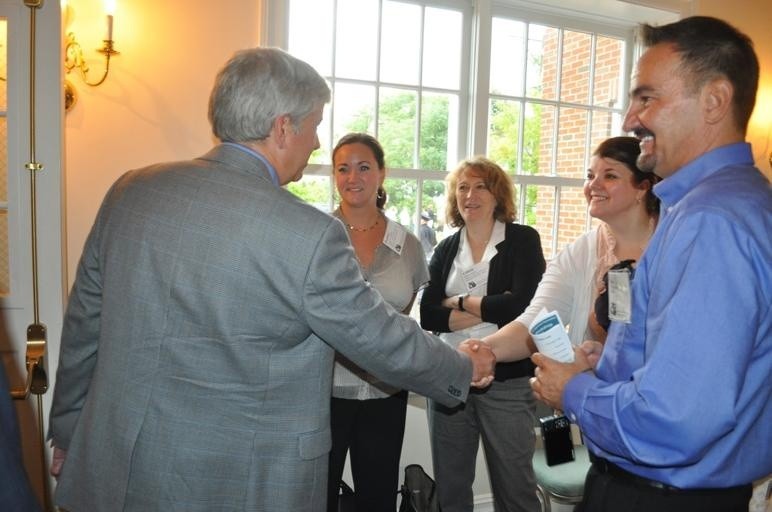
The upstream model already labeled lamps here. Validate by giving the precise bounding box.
[60,1,121,112]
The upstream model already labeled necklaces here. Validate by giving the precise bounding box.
[332,210,382,232]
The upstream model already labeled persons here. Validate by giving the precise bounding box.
[461,137,663,416]
[427,204,434,229]
[385,207,398,222]
[325,132,431,512]
[46,47,497,512]
[400,206,410,229]
[412,207,418,234]
[529,16,772,512]
[421,157,546,512]
[419,214,437,266]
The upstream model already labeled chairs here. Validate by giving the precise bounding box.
[530,399,595,512]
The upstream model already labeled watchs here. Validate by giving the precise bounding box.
[458,292,470,312]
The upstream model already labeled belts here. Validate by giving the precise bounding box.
[582,452,758,497]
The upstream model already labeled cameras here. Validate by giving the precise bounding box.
[540,414,576,467]
